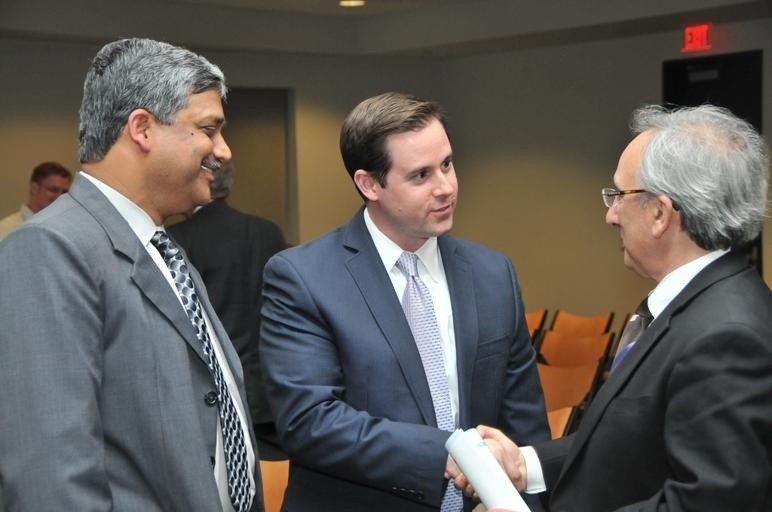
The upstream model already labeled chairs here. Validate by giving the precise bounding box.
[520,310,628,441]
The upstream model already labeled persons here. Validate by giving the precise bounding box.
[438,98,772,511]
[1,35,278,511]
[1,161,75,241]
[143,157,301,464]
[257,88,557,511]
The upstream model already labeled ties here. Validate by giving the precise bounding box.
[395,251,466,512]
[613,290,655,375]
[150,229,255,512]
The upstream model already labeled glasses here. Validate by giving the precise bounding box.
[600,186,683,214]
